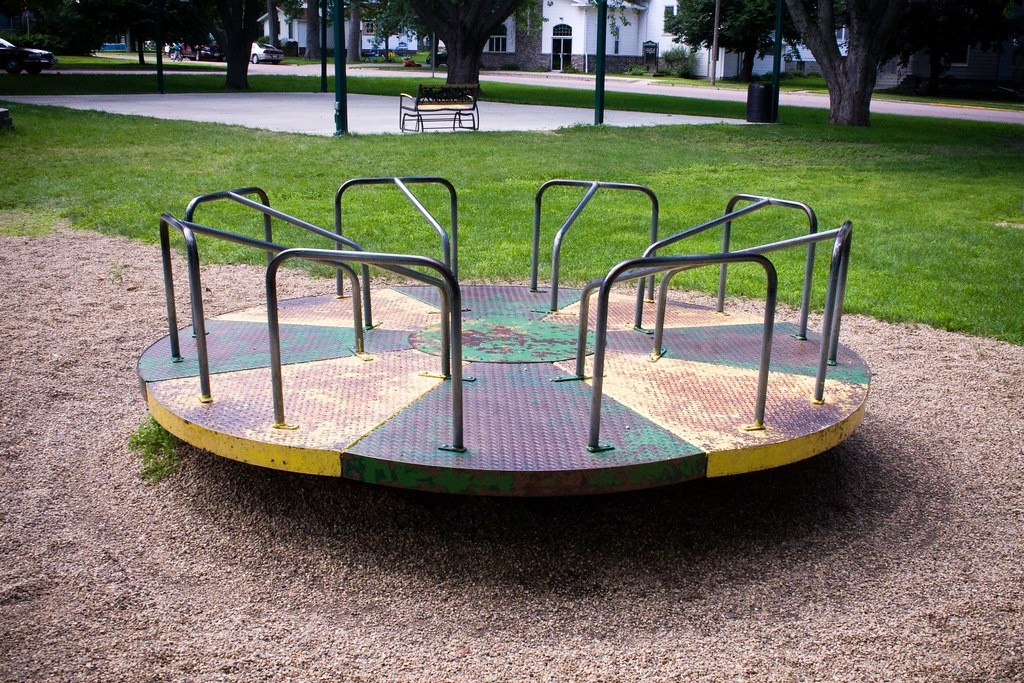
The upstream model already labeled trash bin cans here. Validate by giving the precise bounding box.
[747,82,773,123]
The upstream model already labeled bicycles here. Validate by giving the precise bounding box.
[175,48,183,63]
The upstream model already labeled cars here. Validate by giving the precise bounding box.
[0,38,59,75]
[162,35,226,62]
[395,46,409,57]
[362,50,377,58]
[249,42,284,65]
[425,50,448,69]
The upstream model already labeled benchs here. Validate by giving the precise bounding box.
[400,83,481,133]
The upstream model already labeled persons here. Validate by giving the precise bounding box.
[165,42,183,62]
